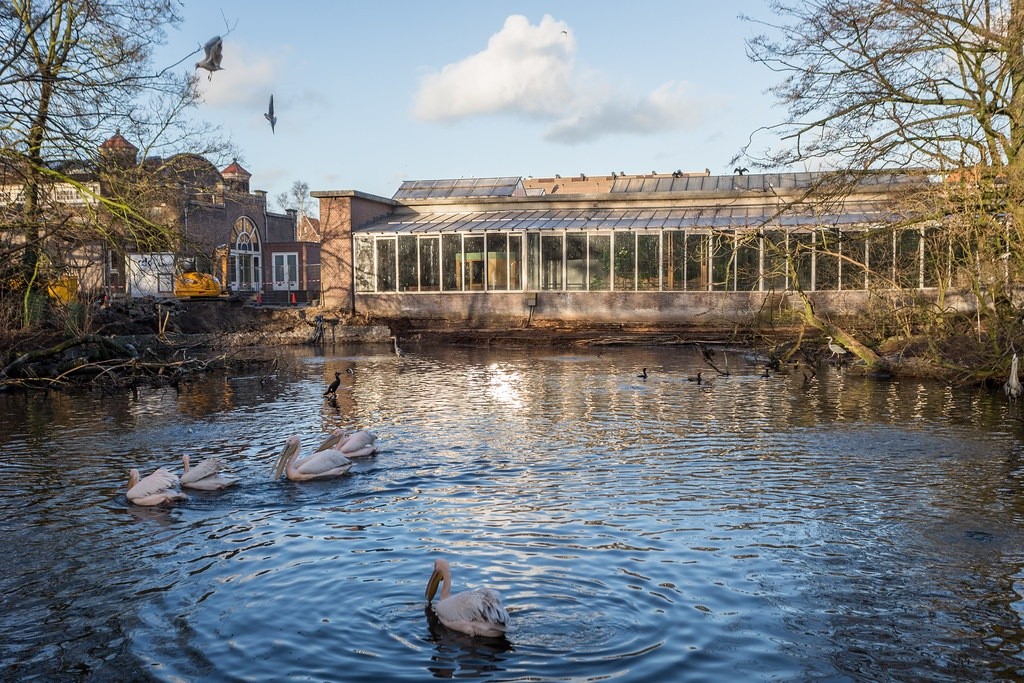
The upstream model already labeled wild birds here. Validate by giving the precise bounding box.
[322,372,341,395]
[389,335,404,357]
[824,336,846,360]
[179,453,241,491]
[426,560,509,638]
[733,165,748,175]
[761,369,771,378]
[125,467,187,506]
[687,373,703,381]
[637,367,648,379]
[264,95,278,133]
[194,36,224,82]
[1002,354,1023,411]
[275,435,357,480]
[702,348,716,362]
[314,428,380,458]
[551,185,558,194]
